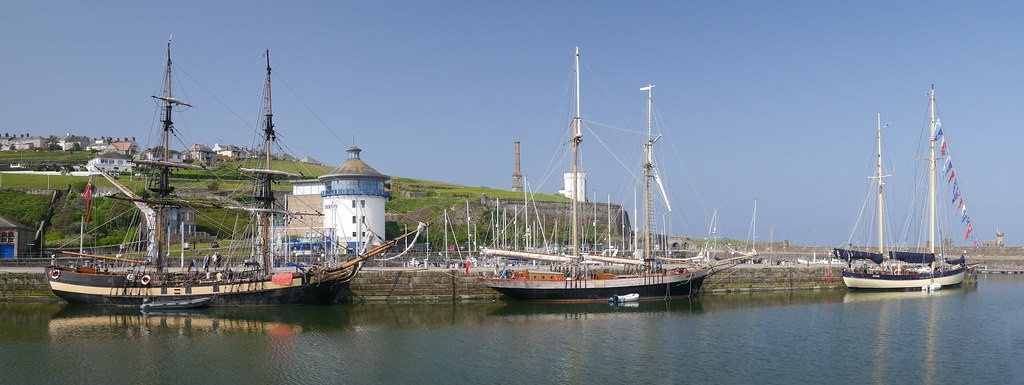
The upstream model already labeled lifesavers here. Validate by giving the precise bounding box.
[517,272,526,280]
[141,275,151,284]
[51,269,61,279]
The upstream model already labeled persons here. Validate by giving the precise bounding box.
[202,251,222,270]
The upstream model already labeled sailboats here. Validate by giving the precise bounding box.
[468,44,762,301]
[438,175,776,269]
[824,82,989,290]
[38,32,434,306]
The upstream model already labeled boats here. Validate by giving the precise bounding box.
[607,292,641,304]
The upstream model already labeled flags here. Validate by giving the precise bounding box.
[84,174,93,222]
